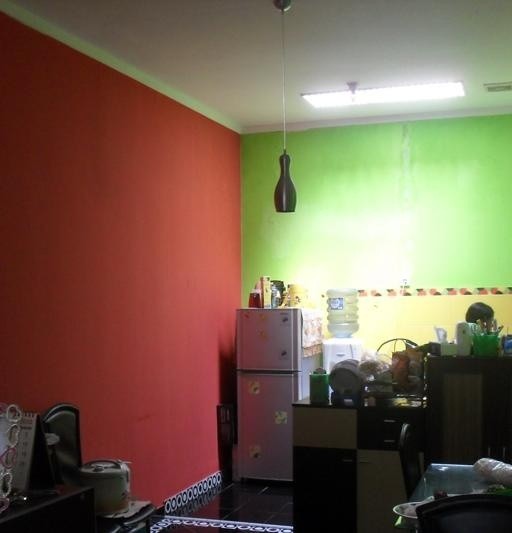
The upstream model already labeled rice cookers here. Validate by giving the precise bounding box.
[77,458,131,514]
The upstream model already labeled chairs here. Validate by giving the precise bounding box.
[416,493,512,532]
[37,402,156,533]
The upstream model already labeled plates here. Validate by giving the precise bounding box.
[328,359,364,393]
[392,501,426,520]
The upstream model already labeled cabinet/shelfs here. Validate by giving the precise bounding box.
[292,403,432,532]
[432,355,512,464]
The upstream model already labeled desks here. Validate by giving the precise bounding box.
[0,485,94,532]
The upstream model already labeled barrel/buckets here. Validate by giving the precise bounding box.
[326,287,359,338]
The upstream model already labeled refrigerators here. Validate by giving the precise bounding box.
[236,308,324,484]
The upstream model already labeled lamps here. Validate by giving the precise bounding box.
[274,0,297,213]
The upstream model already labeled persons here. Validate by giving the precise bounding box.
[464,302,494,334]
